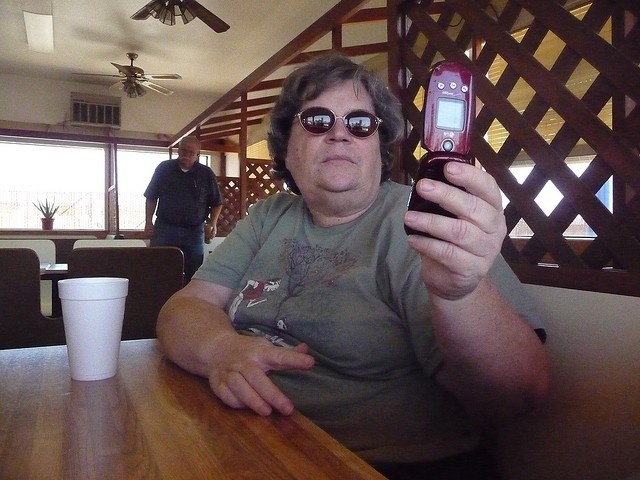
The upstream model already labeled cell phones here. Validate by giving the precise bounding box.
[403,61,476,241]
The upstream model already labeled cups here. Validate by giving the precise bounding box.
[58,277,130,379]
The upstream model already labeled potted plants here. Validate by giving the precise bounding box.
[31,195,64,230]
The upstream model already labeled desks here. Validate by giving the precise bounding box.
[40,261,72,319]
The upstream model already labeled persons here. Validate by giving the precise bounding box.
[143,136,224,288]
[156,51,553,479]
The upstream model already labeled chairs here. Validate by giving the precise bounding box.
[2,239,57,264]
[68,246,184,341]
[1,248,61,332]
[73,239,147,248]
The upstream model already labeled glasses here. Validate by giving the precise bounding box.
[381,138,385,139]
[294,106,383,139]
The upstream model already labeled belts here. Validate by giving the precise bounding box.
[166,221,203,229]
